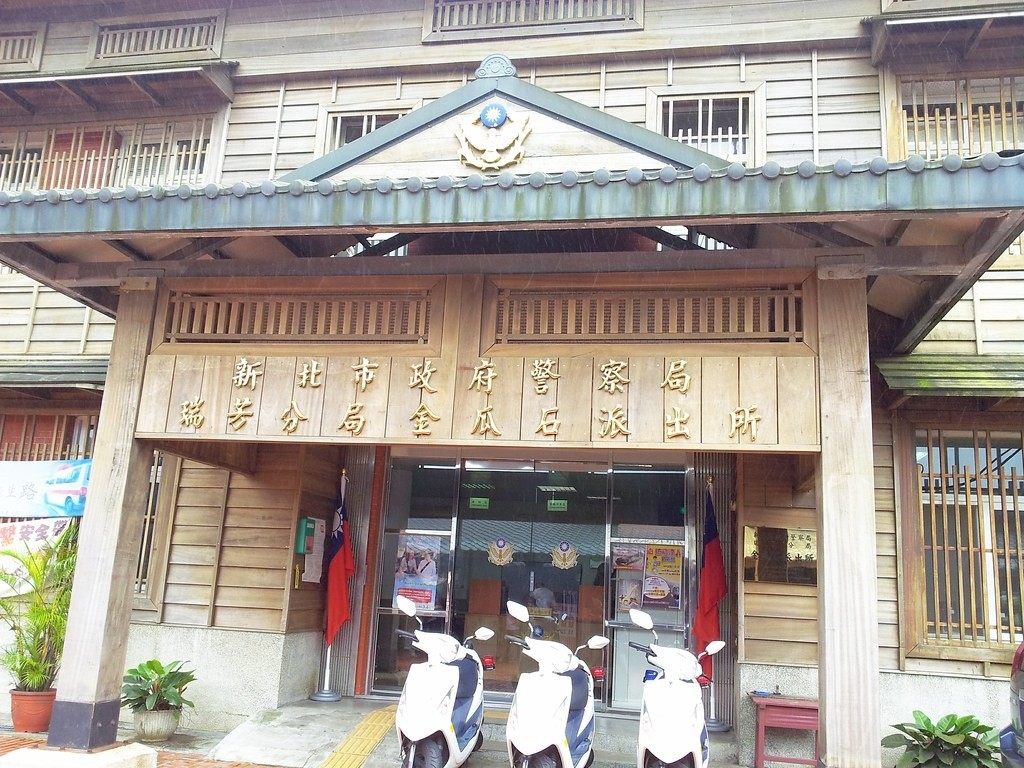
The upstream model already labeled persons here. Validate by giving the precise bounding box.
[414,548,436,576]
[529,580,555,608]
[399,550,417,574]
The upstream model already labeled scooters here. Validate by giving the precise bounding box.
[625,608,726,768]
[391,595,495,768]
[502,600,610,768]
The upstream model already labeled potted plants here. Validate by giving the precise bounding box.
[119,659,198,741]
[0,522,79,733]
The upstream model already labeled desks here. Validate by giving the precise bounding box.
[746,693,820,768]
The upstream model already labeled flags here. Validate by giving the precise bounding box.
[326,474,356,650]
[692,487,729,683]
